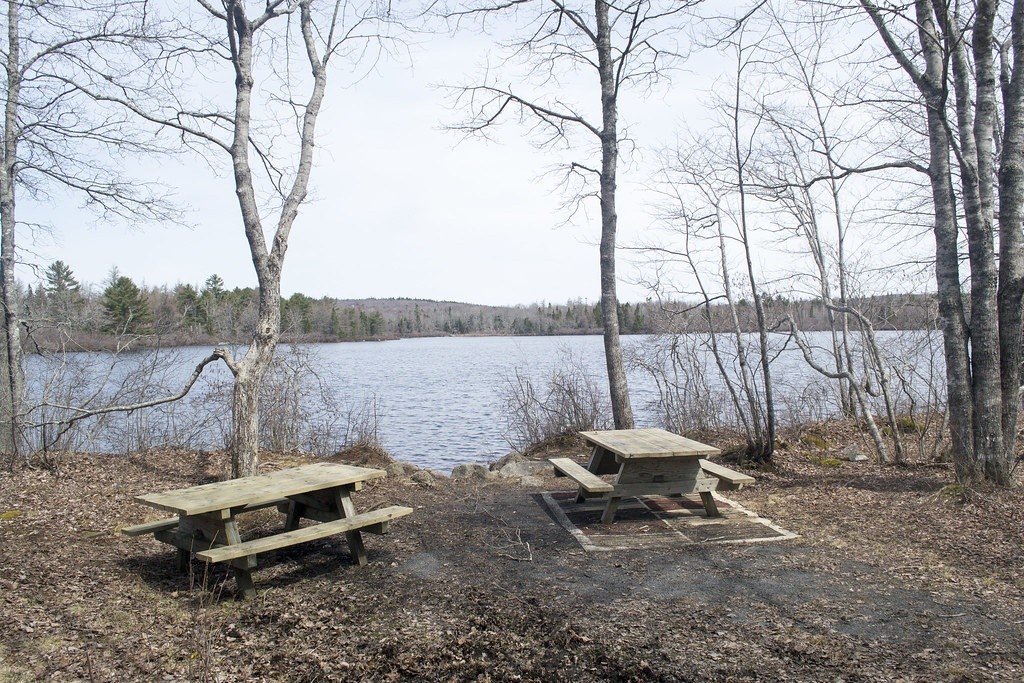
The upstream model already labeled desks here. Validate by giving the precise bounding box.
[134,463,388,602]
[554,428,743,523]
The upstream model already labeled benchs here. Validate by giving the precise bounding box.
[196,505,414,564]
[121,498,290,536]
[698,458,755,484]
[548,457,614,492]
